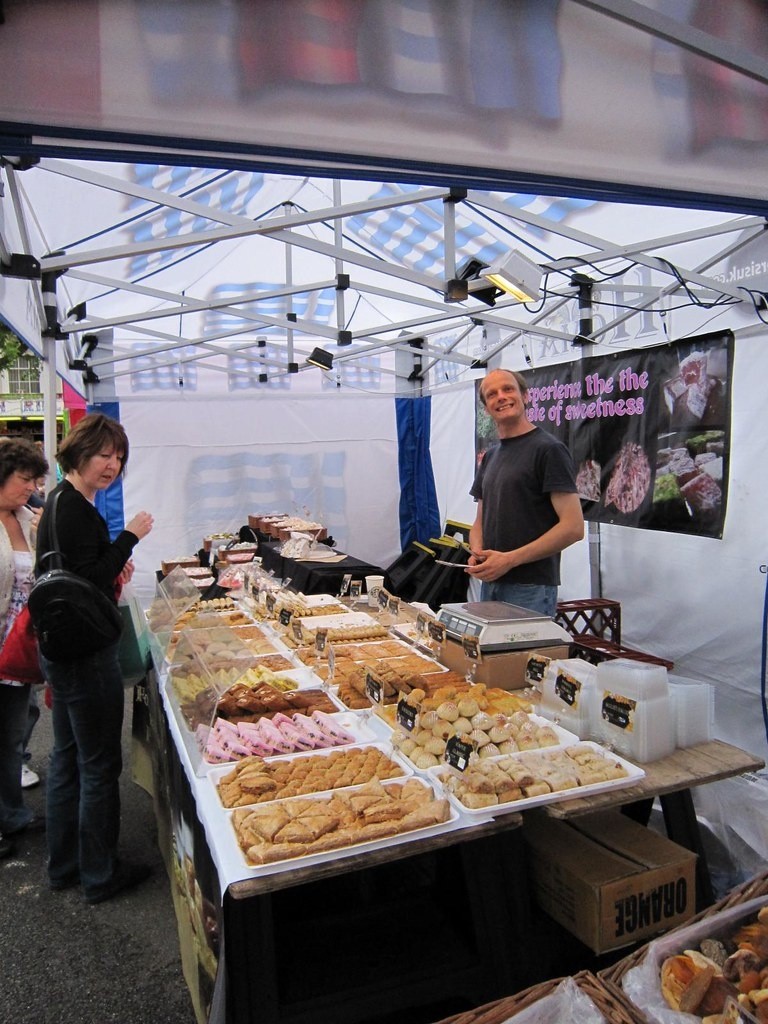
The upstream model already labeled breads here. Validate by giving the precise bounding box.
[661,903,768,1024]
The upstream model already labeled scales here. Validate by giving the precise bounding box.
[433,600,575,654]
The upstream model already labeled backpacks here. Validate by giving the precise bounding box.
[25,488,126,663]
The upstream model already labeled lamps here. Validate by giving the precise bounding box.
[455,256,507,307]
[306,347,333,371]
[444,279,468,303]
[479,248,547,305]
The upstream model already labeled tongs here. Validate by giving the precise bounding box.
[435,534,485,569]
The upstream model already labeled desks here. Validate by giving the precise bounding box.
[260,540,385,596]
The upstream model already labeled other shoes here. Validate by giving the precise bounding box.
[49,860,147,905]
[21,763,40,788]
[0,814,46,856]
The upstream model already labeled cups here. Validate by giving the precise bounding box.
[365,575,384,607]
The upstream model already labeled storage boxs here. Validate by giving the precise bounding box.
[520,811,698,955]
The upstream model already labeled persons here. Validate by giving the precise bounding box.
[464,367,586,621]
[1,437,51,859]
[32,415,157,899]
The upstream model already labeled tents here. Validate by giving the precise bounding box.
[1,0,768,882]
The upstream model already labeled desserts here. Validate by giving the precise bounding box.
[279,537,311,559]
[180,681,451,865]
[576,350,724,518]
[146,591,443,705]
[335,660,629,809]
